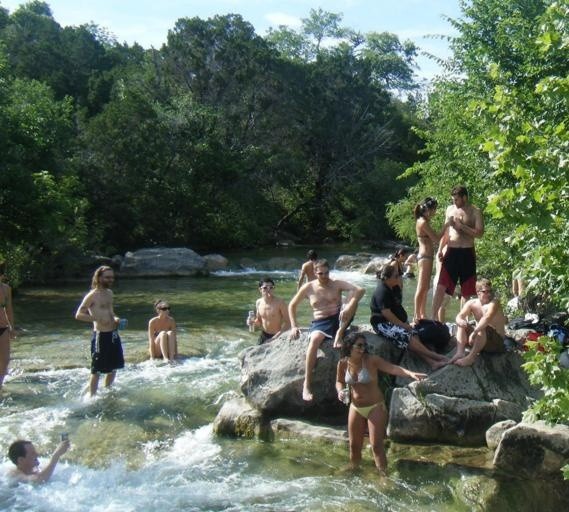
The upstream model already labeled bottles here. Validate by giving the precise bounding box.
[248,309,255,332]
[59,432,68,441]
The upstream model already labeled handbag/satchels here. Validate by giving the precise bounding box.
[414,318,450,349]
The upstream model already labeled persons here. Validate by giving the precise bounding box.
[76,264,126,396]
[147,300,178,361]
[5,438,71,482]
[1,282,19,379]
[245,186,535,467]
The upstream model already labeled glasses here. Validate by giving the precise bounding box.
[105,276,113,279]
[160,307,171,311]
[353,343,368,350]
[317,272,329,275]
[480,290,492,293]
[261,285,275,289]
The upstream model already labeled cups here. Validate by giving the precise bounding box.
[342,390,350,403]
[117,318,127,330]
